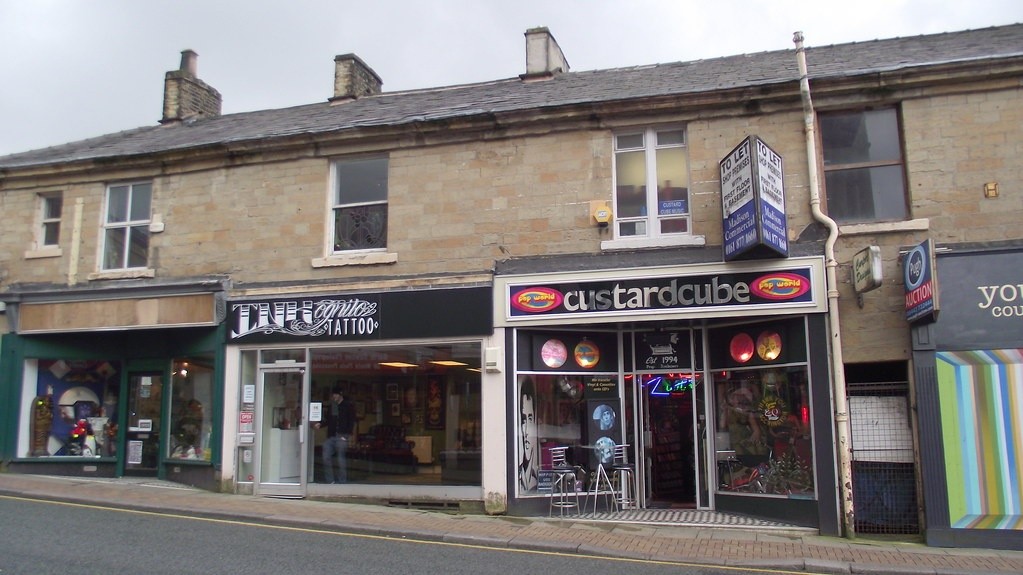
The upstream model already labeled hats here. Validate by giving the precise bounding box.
[331,388,344,396]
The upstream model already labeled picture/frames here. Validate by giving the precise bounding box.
[401,413,412,424]
[401,382,422,411]
[386,383,398,400]
[426,375,447,430]
[273,372,385,428]
[391,402,401,417]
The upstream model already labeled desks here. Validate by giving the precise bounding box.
[581,445,620,519]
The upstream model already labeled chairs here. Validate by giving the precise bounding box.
[609,445,639,515]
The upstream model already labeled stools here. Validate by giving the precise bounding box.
[549,446,581,517]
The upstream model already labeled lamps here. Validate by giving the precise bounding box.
[646,332,671,345]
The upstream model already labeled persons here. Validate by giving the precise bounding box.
[596,440,612,462]
[392,441,419,475]
[719,388,761,466]
[600,405,614,429]
[313,387,356,483]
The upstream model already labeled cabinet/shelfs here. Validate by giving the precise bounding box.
[268,428,314,484]
[406,436,433,465]
